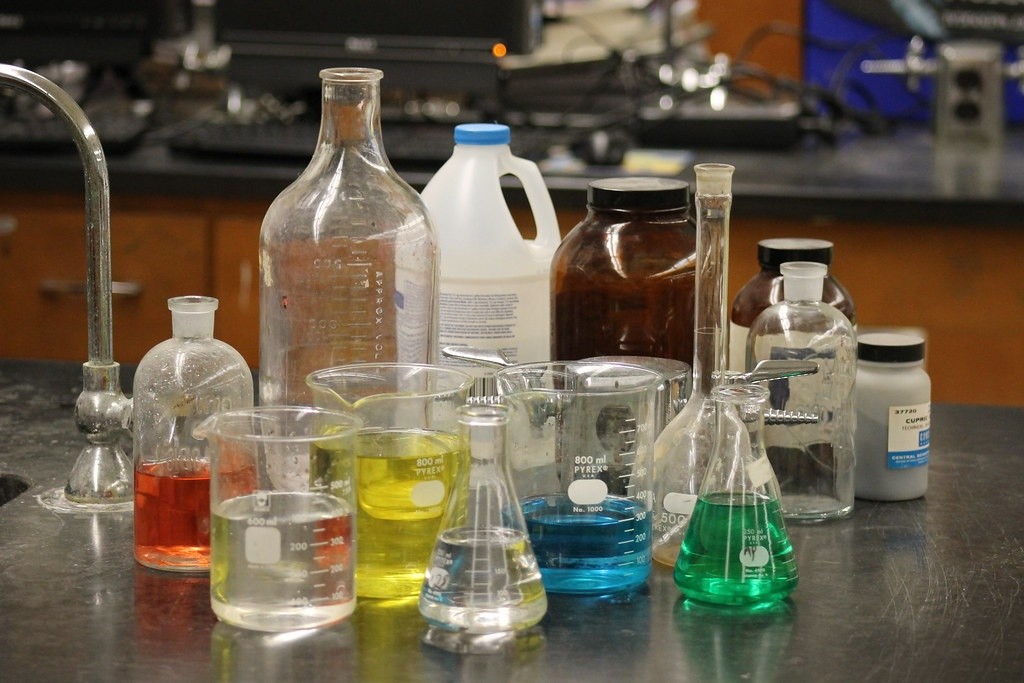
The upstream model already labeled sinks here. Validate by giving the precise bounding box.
[0,468,33,512]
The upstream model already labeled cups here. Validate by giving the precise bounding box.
[193,406,362,631]
[495,363,660,594]
[304,361,473,598]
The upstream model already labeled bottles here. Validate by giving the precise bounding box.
[133,296,253,571]
[257,67,438,407]
[418,123,562,398]
[551,178,697,384]
[855,335,931,499]
[650,163,734,564]
[745,262,854,521]
[673,383,799,603]
[421,405,548,630]
[729,238,858,376]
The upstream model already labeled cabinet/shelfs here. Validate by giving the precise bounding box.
[0,188,220,364]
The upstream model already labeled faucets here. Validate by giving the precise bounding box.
[0,60,132,505]
